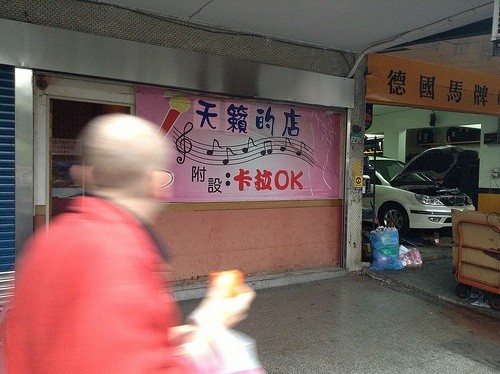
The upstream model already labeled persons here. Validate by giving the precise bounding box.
[0,113,257,374]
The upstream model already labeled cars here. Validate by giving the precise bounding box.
[363,145,480,237]
